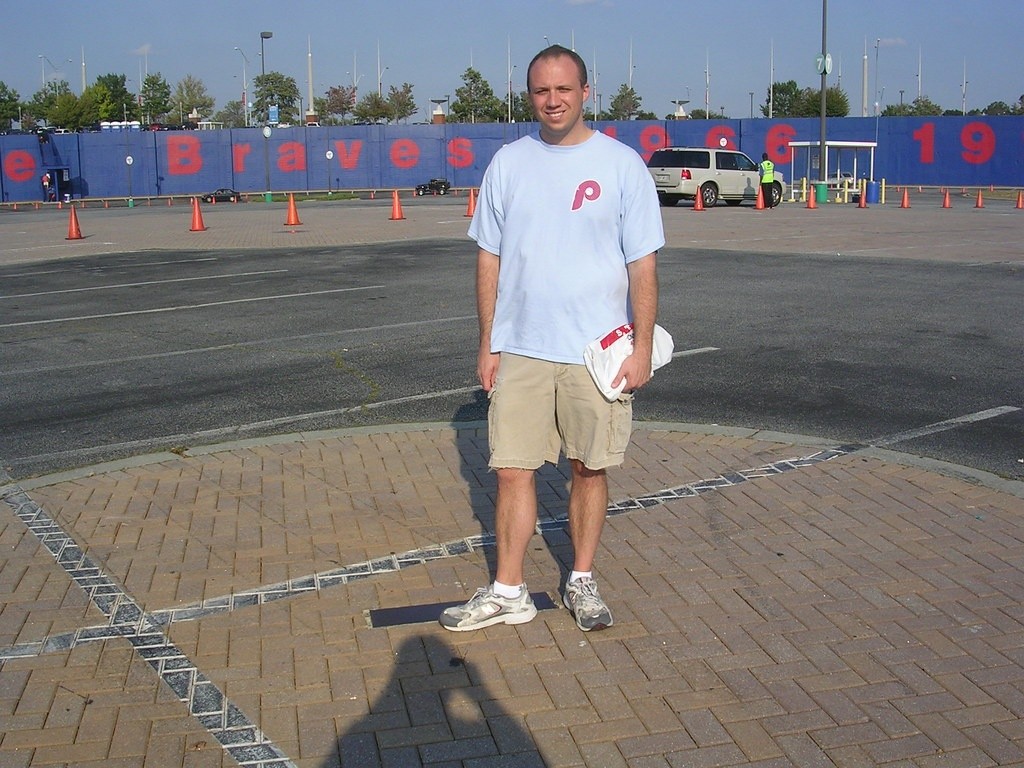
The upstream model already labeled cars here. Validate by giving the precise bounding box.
[0,124,198,133]
[201,188,241,202]
[827,171,853,187]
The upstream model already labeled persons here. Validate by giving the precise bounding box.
[41,172,56,201]
[758,152,775,210]
[439,45,665,631]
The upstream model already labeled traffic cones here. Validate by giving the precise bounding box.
[973,189,985,208]
[691,186,706,211]
[463,189,476,217]
[189,197,207,231]
[856,190,870,208]
[753,186,766,210]
[1014,191,1024,209]
[388,190,407,220]
[940,188,953,208]
[805,185,818,209]
[65,205,86,240]
[898,187,911,208]
[284,193,303,225]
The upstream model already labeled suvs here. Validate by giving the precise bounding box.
[415,179,450,196]
[647,146,787,207]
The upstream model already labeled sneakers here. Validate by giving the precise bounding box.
[439,581,538,631]
[563,571,613,632]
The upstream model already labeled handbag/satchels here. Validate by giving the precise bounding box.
[583,321,675,403]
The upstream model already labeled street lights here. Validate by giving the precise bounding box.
[749,92,755,119]
[260,32,273,126]
